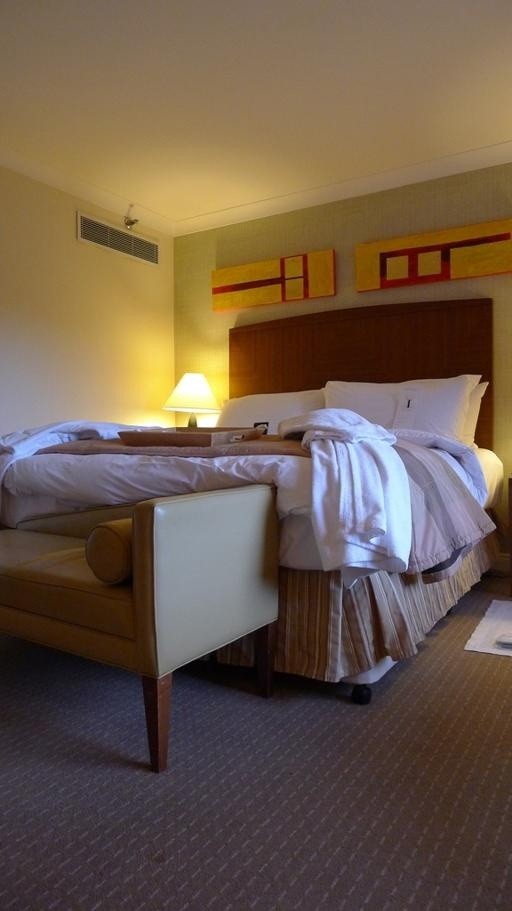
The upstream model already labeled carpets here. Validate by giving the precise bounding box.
[464,597,512,658]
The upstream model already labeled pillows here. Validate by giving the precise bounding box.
[217,388,326,437]
[464,382,490,446]
[323,380,401,432]
[393,375,484,443]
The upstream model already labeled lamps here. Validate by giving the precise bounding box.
[161,372,221,427]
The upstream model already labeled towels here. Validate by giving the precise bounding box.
[278,408,413,588]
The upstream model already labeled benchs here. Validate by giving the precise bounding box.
[0,482,284,772]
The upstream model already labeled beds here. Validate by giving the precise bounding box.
[0,298,505,705]
